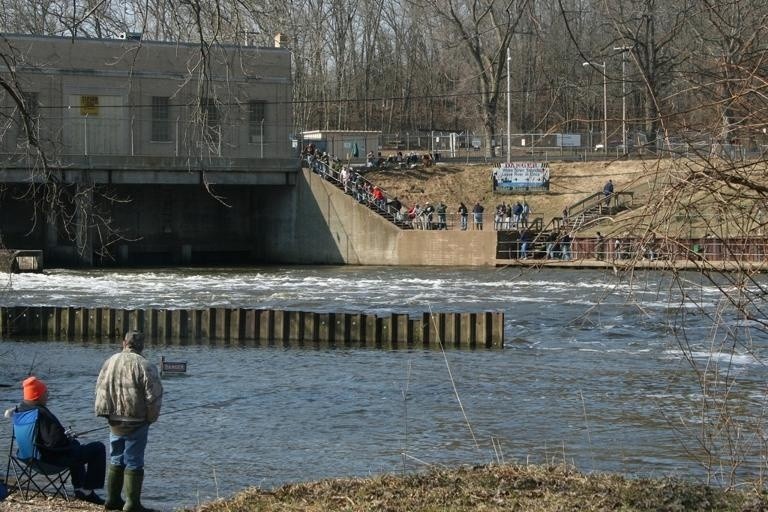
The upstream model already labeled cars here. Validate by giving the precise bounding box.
[376,130,495,152]
[594,137,633,152]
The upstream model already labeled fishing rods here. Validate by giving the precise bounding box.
[74,399,236,443]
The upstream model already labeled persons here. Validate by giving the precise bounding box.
[520,228,658,260]
[4,376,107,504]
[604,180,613,206]
[302,143,529,231]
[94,330,163,512]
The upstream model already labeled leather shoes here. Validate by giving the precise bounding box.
[70,487,107,506]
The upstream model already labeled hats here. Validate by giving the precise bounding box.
[424,202,431,205]
[22,376,48,401]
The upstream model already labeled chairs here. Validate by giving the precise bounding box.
[5,404,72,502]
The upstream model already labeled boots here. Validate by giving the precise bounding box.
[103,463,145,512]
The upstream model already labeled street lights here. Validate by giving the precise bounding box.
[505,46,513,164]
[580,60,608,155]
